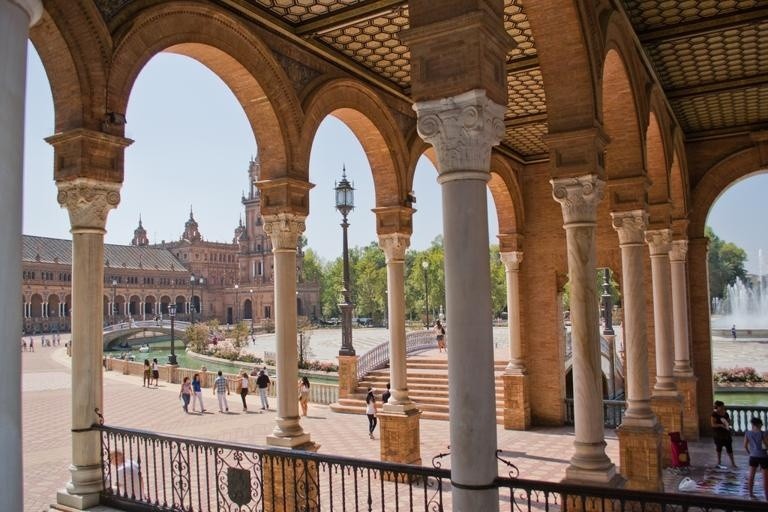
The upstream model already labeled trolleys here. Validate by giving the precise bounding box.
[667,430,691,476]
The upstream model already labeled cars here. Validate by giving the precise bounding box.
[328,315,374,326]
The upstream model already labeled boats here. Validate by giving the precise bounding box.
[139,346,150,353]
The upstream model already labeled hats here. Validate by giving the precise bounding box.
[367,387,376,393]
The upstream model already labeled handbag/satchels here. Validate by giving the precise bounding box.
[298,393,303,400]
[762,439,768,450]
[236,383,243,394]
[441,328,446,335]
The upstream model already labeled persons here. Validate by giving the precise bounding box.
[212,336,217,345]
[191,374,206,413]
[118,341,148,360]
[149,358,159,386]
[107,448,146,502]
[251,370,270,409]
[142,358,150,388]
[732,324,737,342]
[251,334,256,345]
[178,376,193,413]
[381,382,390,404]
[21,327,61,352]
[743,417,768,497]
[710,399,738,467]
[297,375,309,416]
[365,388,378,438]
[432,320,447,353]
[250,368,257,377]
[233,372,253,411]
[212,370,230,413]
[262,367,269,376]
[153,315,162,326]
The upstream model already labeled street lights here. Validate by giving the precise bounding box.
[248,288,255,320]
[199,274,205,321]
[110,273,119,323]
[167,300,178,365]
[296,325,305,369]
[191,303,194,323]
[382,289,388,329]
[188,273,196,321]
[233,282,240,324]
[333,163,359,394]
[420,257,433,331]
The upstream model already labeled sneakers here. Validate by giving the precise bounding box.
[225,408,229,412]
[260,405,270,411]
[369,432,375,439]
